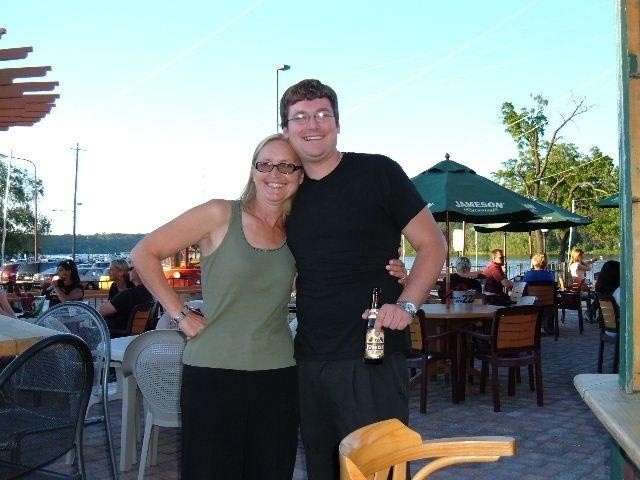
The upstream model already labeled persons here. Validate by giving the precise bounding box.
[0,285,17,319]
[482,249,514,306]
[521,254,555,281]
[437,257,482,303]
[280,79,449,480]
[107,259,136,301]
[130,133,407,480]
[30,259,84,317]
[568,249,604,324]
[98,265,158,383]
[595,260,620,338]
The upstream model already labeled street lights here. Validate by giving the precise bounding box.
[541,229,549,254]
[0,152,38,262]
[274,65,291,138]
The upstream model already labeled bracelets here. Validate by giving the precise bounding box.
[174,304,190,324]
[53,285,59,288]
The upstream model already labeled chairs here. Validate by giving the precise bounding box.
[0,332,97,478]
[336,418,514,479]
[116,325,187,478]
[408,272,620,416]
[1,275,206,363]
[32,300,121,479]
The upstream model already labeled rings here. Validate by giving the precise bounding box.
[401,274,405,280]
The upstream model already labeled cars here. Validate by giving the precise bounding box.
[0,252,128,290]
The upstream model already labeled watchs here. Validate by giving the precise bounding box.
[397,300,418,318]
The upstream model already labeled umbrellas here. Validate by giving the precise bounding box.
[473,196,595,259]
[410,153,555,302]
[595,193,619,208]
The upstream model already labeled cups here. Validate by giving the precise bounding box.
[42,300,49,313]
[67,306,77,316]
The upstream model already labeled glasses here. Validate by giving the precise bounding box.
[289,111,335,122]
[255,161,303,174]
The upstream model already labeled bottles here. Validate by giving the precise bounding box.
[362,287,385,364]
